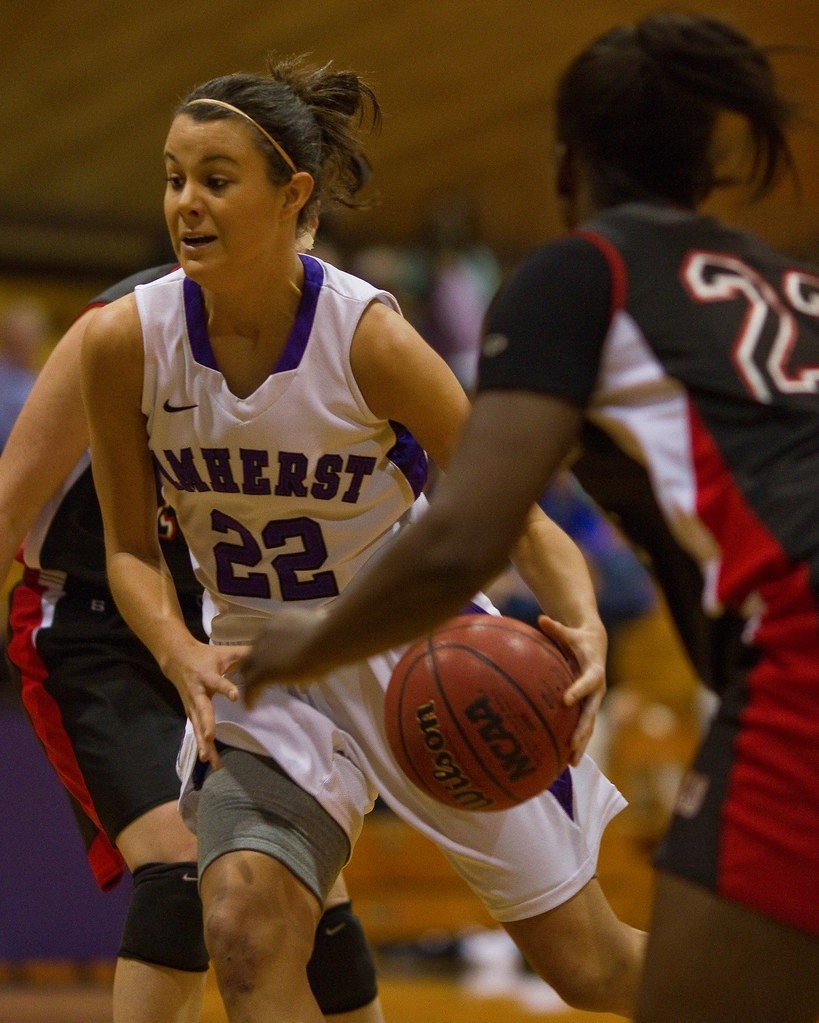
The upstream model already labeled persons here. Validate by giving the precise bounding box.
[208,13,819,1022]
[0,193,724,1022]
[78,56,655,1023]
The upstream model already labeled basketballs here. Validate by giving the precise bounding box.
[382,613,587,812]
